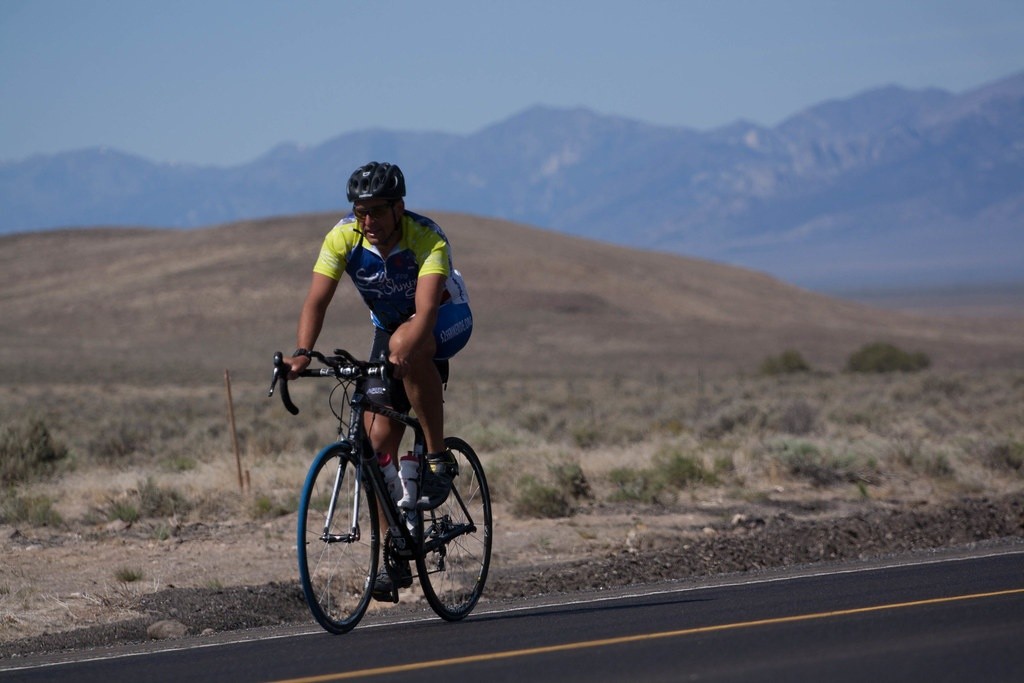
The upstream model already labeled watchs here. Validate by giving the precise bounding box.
[292,348,312,366]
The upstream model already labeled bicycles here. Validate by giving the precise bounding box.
[269,352,493,635]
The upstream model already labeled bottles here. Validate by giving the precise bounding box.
[376,450,404,502]
[397,450,420,510]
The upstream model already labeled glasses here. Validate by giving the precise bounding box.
[352,196,400,219]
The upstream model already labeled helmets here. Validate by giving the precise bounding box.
[346,161,407,201]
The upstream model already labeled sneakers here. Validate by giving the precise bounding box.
[363,562,413,596]
[416,449,459,511]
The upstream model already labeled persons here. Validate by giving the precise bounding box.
[287,161,473,595]
